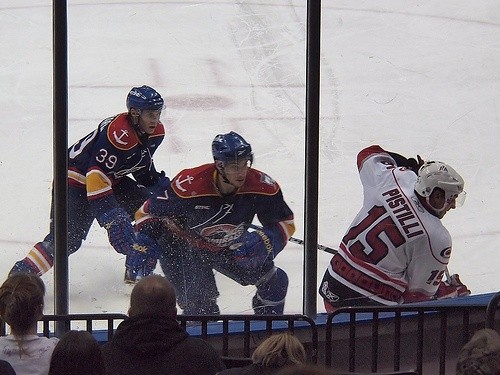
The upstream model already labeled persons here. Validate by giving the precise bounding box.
[0,273,353,375]
[318,145,476,314]
[1,86,176,296]
[135,132,296,325]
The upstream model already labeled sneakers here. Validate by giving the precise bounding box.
[122,268,139,293]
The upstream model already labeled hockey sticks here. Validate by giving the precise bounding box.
[244,220,339,256]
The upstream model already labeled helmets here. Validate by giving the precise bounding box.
[127,85,165,113]
[414,161,464,215]
[212,131,253,163]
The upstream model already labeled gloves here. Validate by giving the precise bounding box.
[228,227,285,274]
[126,234,161,276]
[436,274,471,301]
[97,207,138,256]
